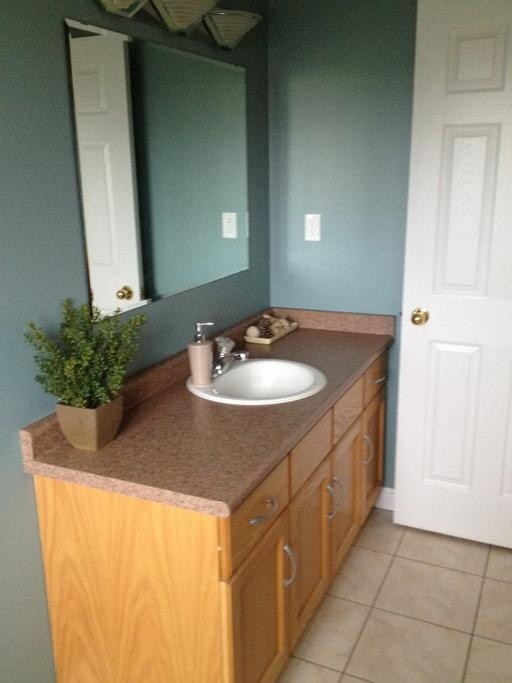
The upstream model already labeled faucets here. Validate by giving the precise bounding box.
[210,334,251,380]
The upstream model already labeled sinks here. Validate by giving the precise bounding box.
[185,356,328,406]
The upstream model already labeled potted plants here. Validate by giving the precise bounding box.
[23,297,145,454]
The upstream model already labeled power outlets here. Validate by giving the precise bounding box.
[222,211,237,239]
[305,213,321,240]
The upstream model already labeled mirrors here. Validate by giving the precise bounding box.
[63,16,250,322]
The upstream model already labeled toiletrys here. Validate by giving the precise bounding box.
[187,321,216,387]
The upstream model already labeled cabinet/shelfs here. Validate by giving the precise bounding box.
[333,373,364,585]
[362,356,384,529]
[34,450,290,683]
[291,408,333,654]
